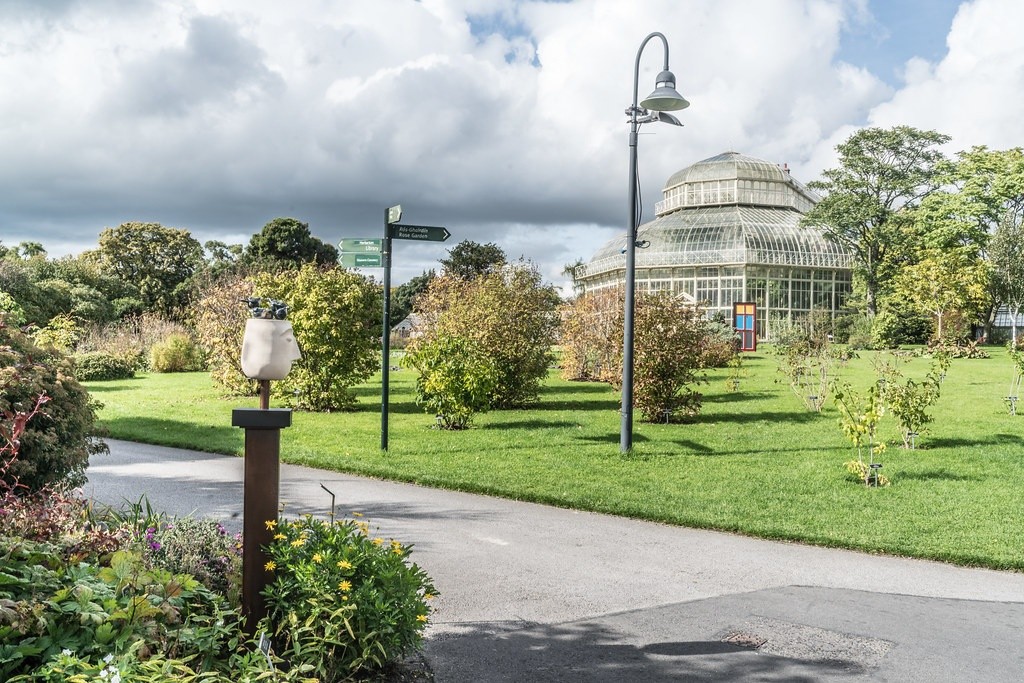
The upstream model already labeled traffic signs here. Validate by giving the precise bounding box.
[336,254,383,269]
[337,237,382,252]
[387,204,403,225]
[391,225,450,241]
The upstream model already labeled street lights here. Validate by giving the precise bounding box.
[619,31,689,457]
